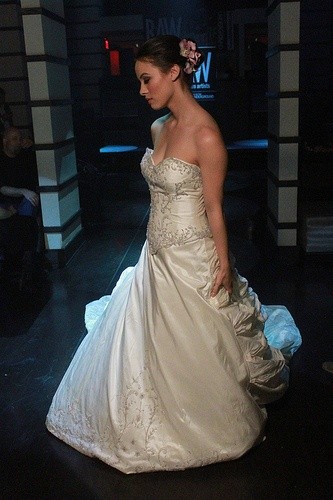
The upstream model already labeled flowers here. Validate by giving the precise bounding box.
[179,39,201,74]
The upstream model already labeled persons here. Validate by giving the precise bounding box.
[0,127,43,254]
[44,35,302,475]
[0,203,53,338]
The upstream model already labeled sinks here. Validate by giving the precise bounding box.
[230,138,268,148]
[98,144,139,153]
